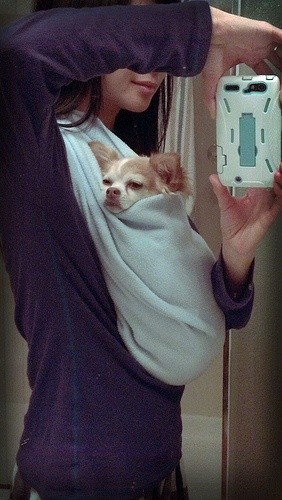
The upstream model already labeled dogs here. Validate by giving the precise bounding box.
[87,139,195,214]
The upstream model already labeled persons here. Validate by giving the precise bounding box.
[1,0,281,498]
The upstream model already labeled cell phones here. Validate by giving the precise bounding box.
[214,74,282,188]
